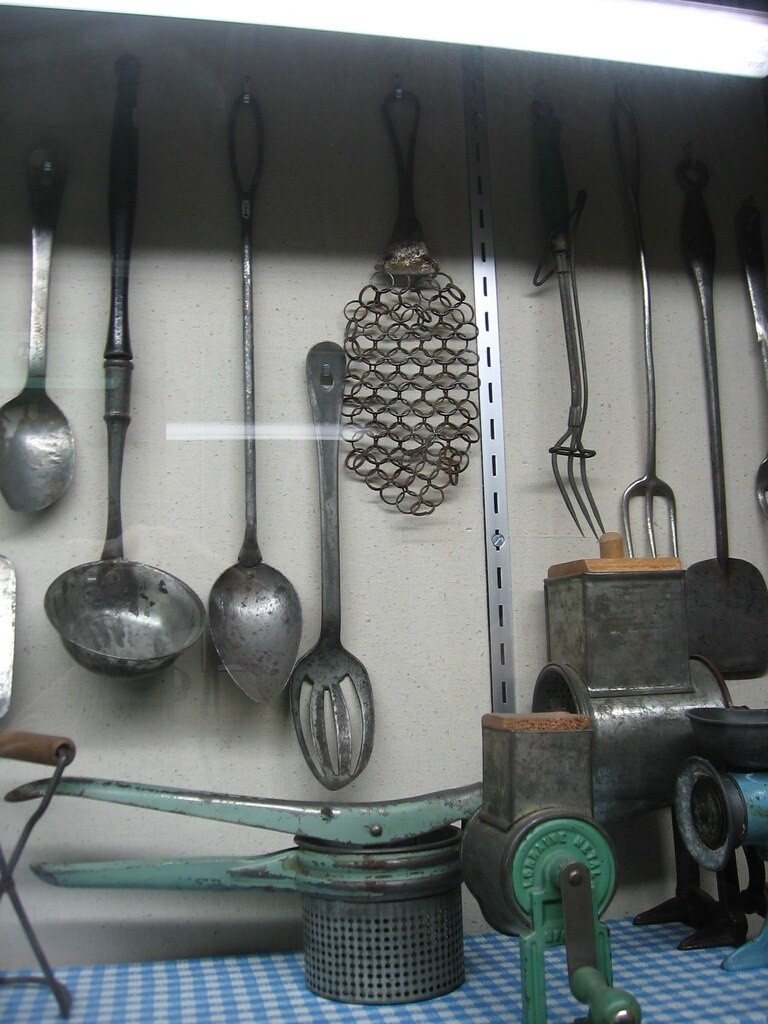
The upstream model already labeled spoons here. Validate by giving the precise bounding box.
[0,140,76,515]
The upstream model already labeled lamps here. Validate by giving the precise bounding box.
[0,0,768,79]
